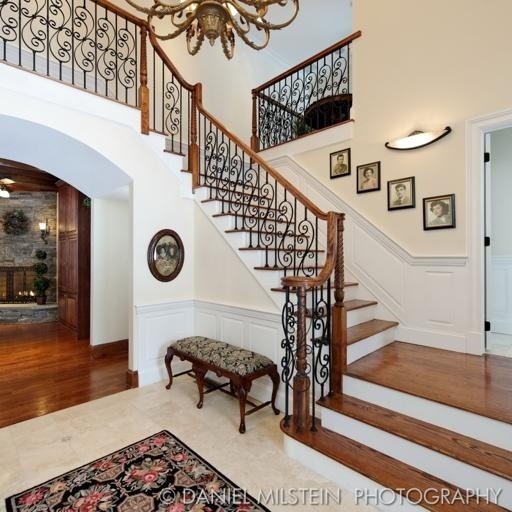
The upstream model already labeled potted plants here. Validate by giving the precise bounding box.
[32,250,49,304]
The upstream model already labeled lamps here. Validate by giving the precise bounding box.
[38,222,50,245]
[125,0,299,59]
[385,126,452,151]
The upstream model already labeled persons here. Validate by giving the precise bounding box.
[360,168,378,188]
[393,184,410,204]
[334,154,347,174]
[154,245,167,261]
[428,200,451,226]
[168,245,178,260]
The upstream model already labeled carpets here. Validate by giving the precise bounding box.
[6,430,272,512]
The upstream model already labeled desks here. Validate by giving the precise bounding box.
[304,94,352,130]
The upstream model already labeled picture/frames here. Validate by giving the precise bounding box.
[357,161,380,193]
[147,229,184,282]
[330,148,351,178]
[423,194,455,230]
[387,177,415,210]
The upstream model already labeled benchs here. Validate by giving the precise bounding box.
[165,336,280,433]
